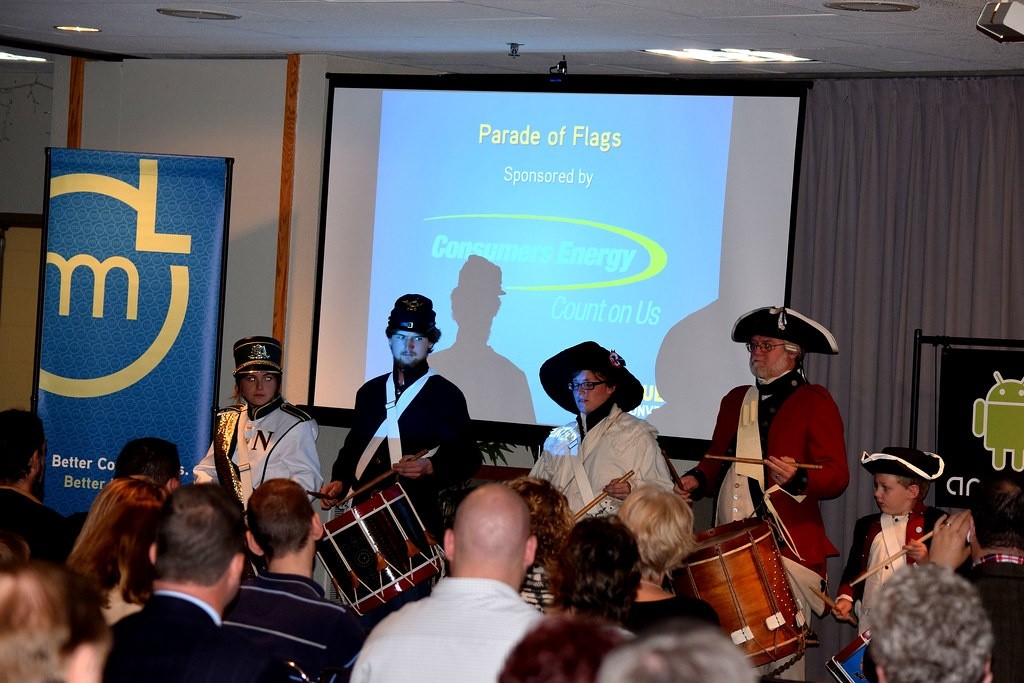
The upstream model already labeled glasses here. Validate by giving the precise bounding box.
[568,382,608,391]
[745,339,796,355]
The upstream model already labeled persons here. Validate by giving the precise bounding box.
[869,474,1024,683]
[0,409,365,683]
[320,294,482,635]
[351,476,759,683]
[673,307,850,683]
[830,446,949,637]
[524,340,673,524]
[193,336,323,503]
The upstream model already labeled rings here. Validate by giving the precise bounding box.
[775,473,780,478]
[945,522,951,526]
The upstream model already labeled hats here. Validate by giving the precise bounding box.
[859,446,947,481]
[539,341,643,414]
[231,336,284,376]
[731,310,839,354]
[386,294,440,343]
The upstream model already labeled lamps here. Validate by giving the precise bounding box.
[976,0,1024,42]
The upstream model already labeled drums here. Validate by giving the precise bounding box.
[668,520,809,667]
[826,626,874,683]
[312,480,446,614]
